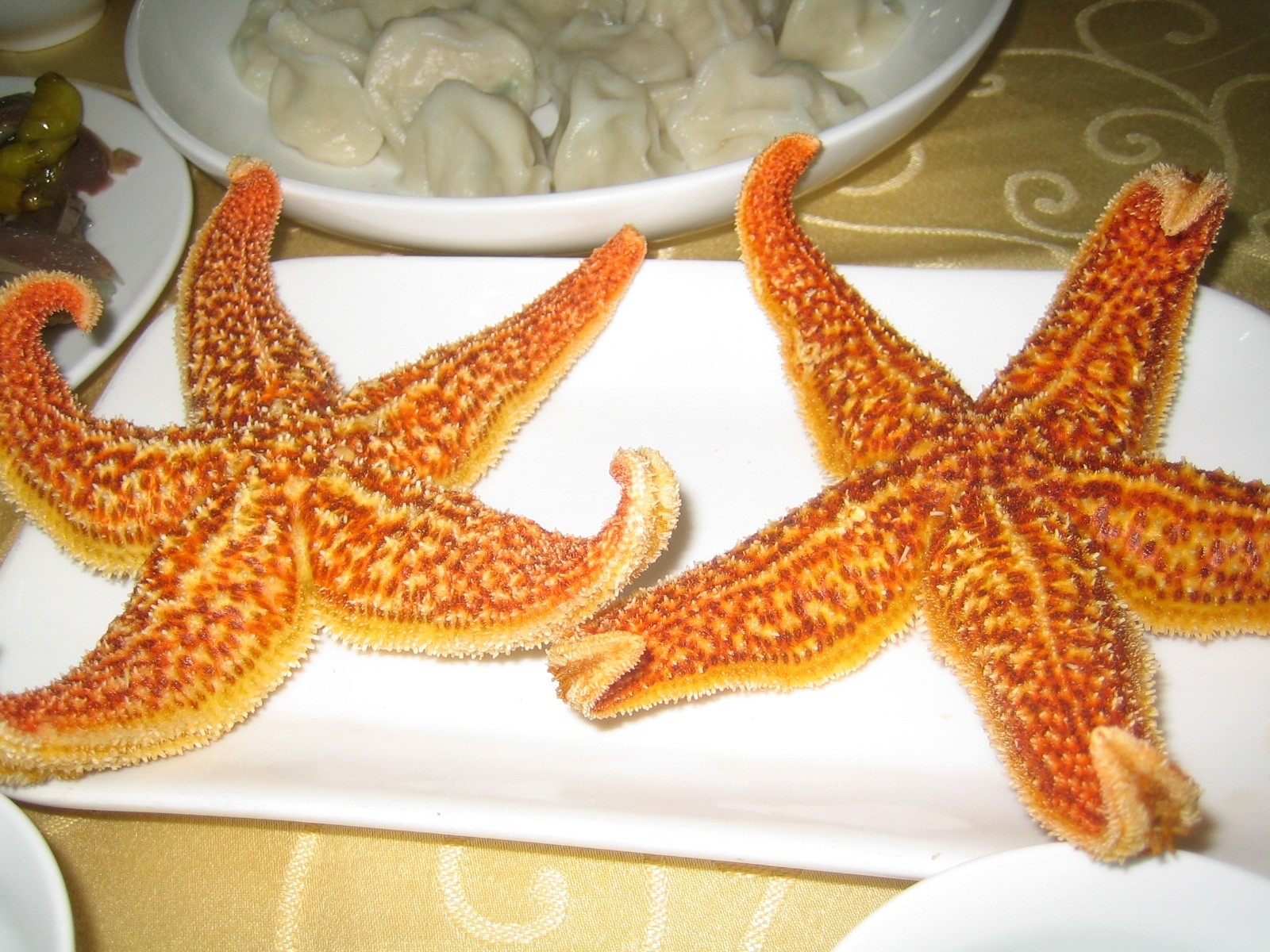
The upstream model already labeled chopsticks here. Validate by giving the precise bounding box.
[0,793,75,952]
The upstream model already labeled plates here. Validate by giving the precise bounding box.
[0,256,1269,879]
[0,74,193,392]
[832,842,1269,952]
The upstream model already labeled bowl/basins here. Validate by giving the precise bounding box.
[125,0,1013,254]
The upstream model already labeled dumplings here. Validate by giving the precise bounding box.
[221,0,913,199]
